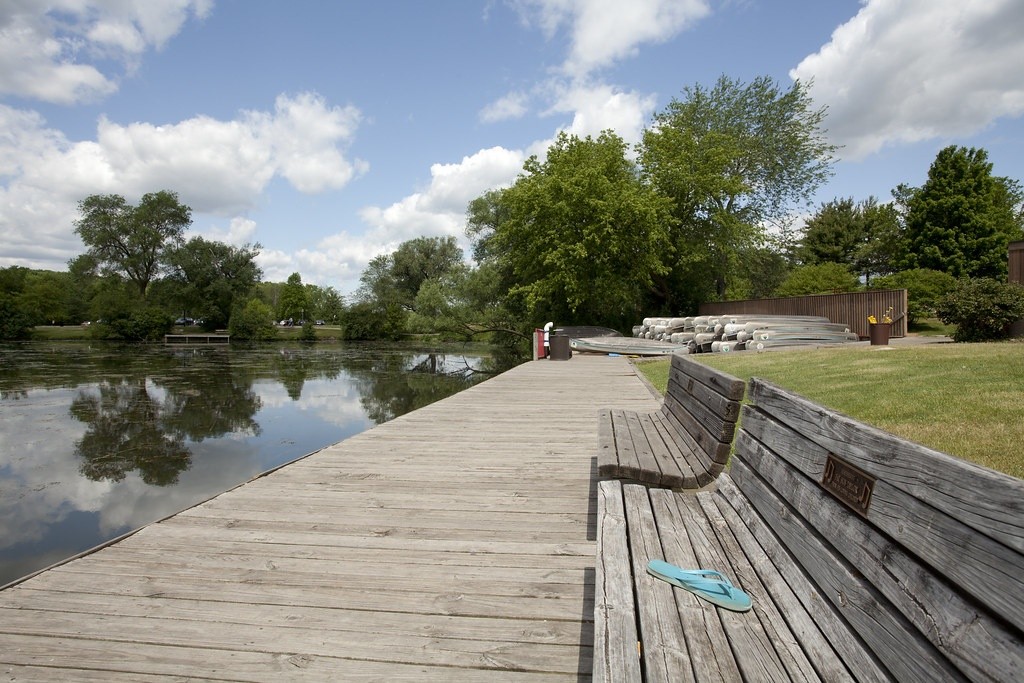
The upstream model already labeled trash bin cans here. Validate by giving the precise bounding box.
[549,329,573,362]
[869,323,890,345]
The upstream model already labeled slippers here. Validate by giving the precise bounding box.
[646,559,752,612]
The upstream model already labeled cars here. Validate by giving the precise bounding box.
[298,319,306,325]
[313,319,325,326]
[81,321,91,327]
[194,318,206,326]
[175,315,194,324]
[96,318,103,324]
[280,320,289,325]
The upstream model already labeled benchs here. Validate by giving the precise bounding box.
[596,353,745,490]
[592,376,1024,683]
